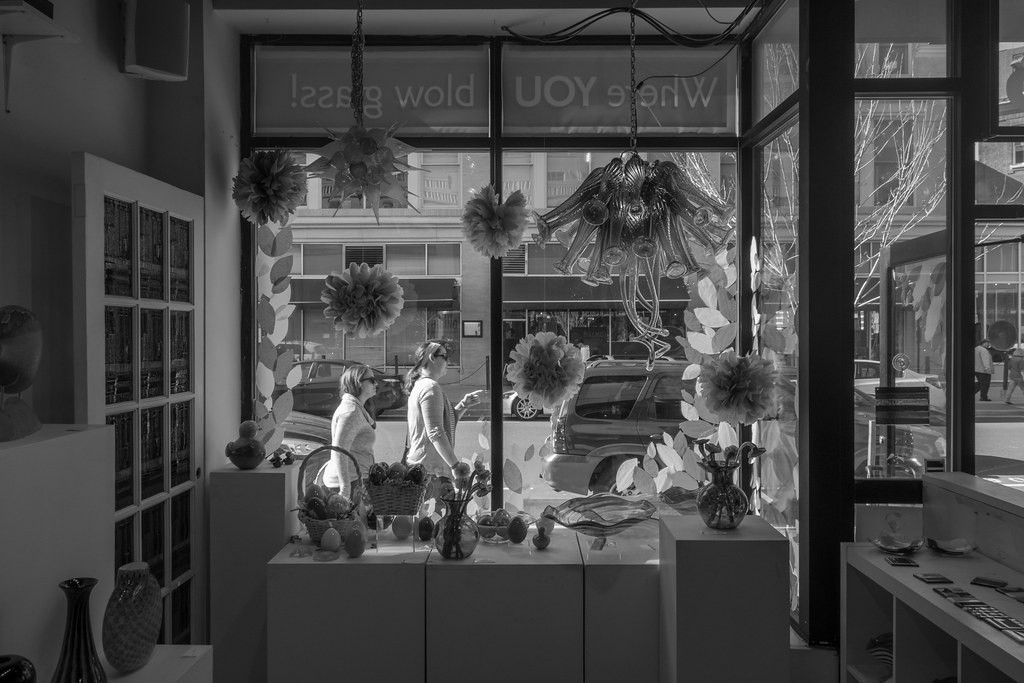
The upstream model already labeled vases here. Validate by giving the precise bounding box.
[49,576,108,683]
[102,560,163,672]
[432,493,481,561]
[695,461,748,530]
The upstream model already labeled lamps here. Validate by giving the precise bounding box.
[529,0,737,289]
[301,0,432,227]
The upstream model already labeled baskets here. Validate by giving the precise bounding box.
[297,445,362,543]
[363,479,426,515]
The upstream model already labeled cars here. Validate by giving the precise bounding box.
[502,390,553,422]
[272,358,407,418]
[854,356,945,423]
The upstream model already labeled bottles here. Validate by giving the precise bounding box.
[48,577,110,683]
[102,561,163,672]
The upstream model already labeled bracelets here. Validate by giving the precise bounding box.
[451,462,460,468]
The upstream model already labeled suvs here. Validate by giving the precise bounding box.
[543,357,946,495]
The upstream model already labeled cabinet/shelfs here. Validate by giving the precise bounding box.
[839,541,1024,683]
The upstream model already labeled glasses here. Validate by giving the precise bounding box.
[359,377,377,385]
[435,353,448,361]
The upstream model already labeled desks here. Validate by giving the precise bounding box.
[208,460,306,683]
[658,515,789,683]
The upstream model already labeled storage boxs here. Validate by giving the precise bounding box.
[576,519,660,683]
[425,528,584,683]
[265,528,435,683]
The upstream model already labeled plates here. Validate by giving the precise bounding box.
[927,537,977,554]
[867,531,925,555]
[865,632,894,673]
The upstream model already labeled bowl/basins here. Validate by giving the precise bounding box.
[475,511,538,543]
[543,496,656,536]
[659,487,707,509]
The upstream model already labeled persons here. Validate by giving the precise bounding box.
[1005,350,1024,405]
[404,344,465,481]
[974,339,994,401]
[322,365,379,502]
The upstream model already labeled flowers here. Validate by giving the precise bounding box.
[505,330,586,409]
[692,438,767,465]
[230,145,310,227]
[459,180,531,260]
[699,350,780,427]
[438,460,493,500]
[320,260,406,340]
